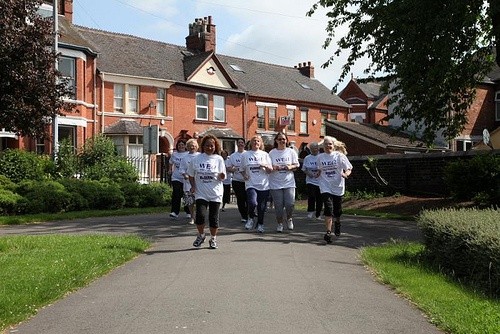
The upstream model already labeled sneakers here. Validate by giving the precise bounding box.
[241,216,247,222]
[256,223,264,232]
[245,218,254,230]
[209,239,217,248]
[169,212,178,219]
[186,214,191,218]
[334,222,341,236]
[189,218,194,225]
[276,222,283,232]
[307,211,315,220]
[287,217,294,229]
[324,232,332,243]
[193,233,206,247]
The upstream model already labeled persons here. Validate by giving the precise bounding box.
[239,136,273,233]
[334,140,347,155]
[227,138,249,222]
[314,136,353,244]
[180,139,198,225]
[187,134,226,248]
[221,151,231,211]
[302,142,323,220]
[169,140,191,218]
[268,133,300,233]
